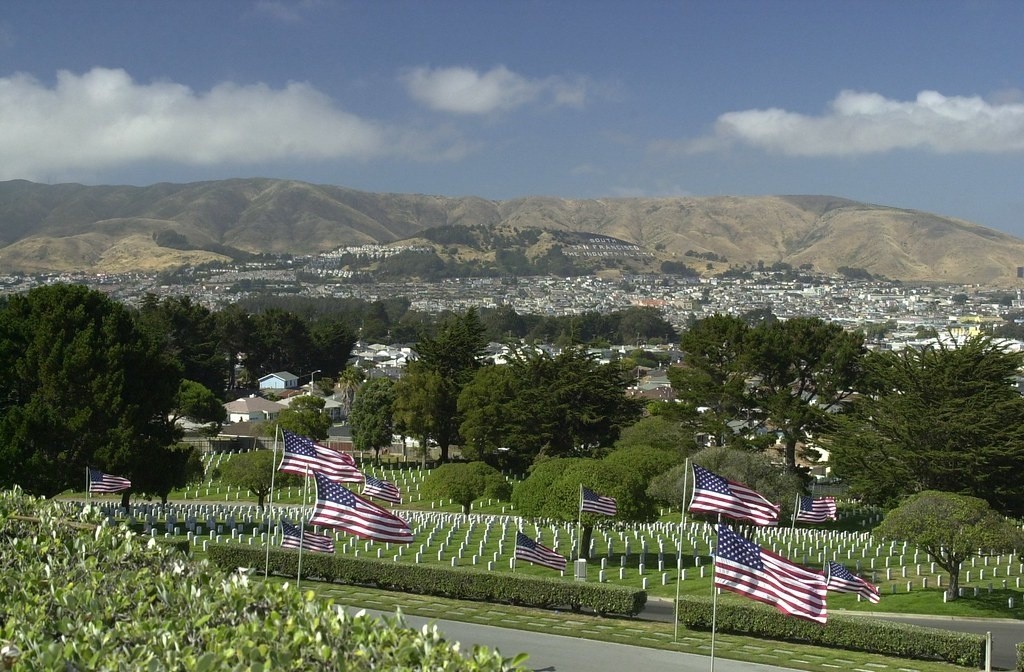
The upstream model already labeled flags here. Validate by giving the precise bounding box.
[361,474,400,504]
[581,486,617,516]
[279,519,334,553]
[515,533,567,571]
[796,496,837,523]
[714,524,828,624]
[308,470,413,544]
[277,428,363,483]
[88,469,131,493]
[826,559,880,603]
[688,463,781,526]
